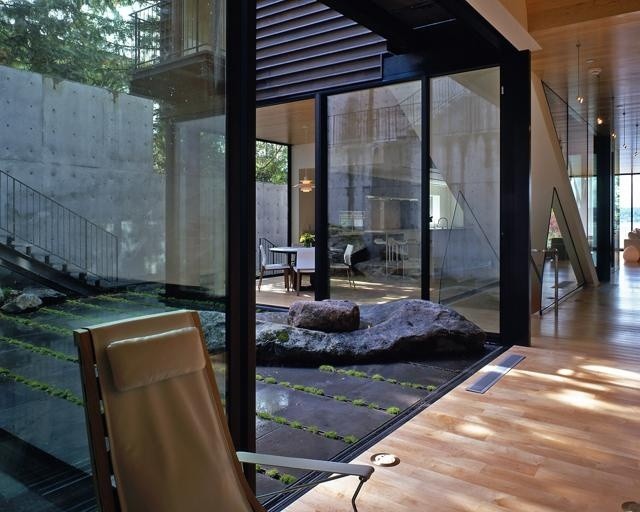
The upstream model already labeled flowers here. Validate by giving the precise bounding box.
[298,232,316,247]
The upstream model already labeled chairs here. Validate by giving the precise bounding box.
[258,243,356,296]
[72,310,376,511]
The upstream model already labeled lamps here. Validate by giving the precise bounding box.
[575,43,639,157]
[292,127,316,193]
